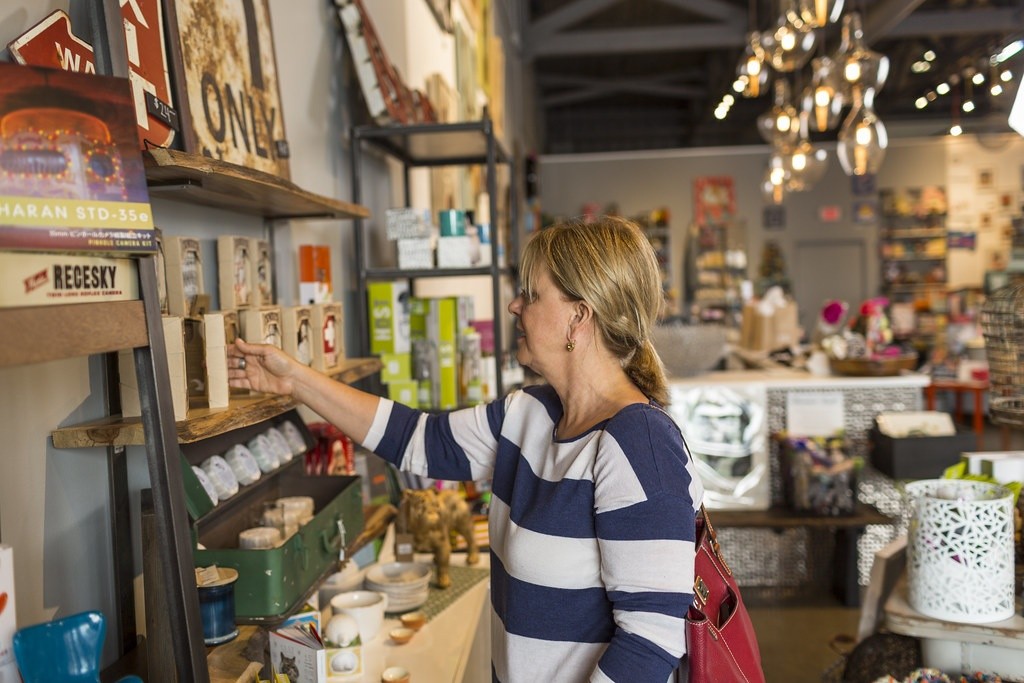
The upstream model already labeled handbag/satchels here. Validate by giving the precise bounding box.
[773,429,865,517]
[617,403,766,683]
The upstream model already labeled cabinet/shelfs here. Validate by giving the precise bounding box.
[204,548,496,683]
[51,147,400,683]
[881,188,949,303]
[883,558,1024,682]
[697,505,892,682]
[343,105,526,409]
[617,203,752,322]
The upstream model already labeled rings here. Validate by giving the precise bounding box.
[239,358,244,369]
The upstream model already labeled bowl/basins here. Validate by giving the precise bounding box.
[401,614,426,631]
[319,574,365,609]
[330,590,387,642]
[382,667,411,683]
[390,629,413,644]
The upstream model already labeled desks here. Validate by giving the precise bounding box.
[925,383,990,430]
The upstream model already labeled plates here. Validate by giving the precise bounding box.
[366,562,432,613]
[830,356,917,376]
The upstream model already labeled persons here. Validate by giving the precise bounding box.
[228,215,704,683]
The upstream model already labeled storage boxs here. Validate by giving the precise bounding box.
[869,426,975,481]
[157,410,364,626]
[365,203,482,407]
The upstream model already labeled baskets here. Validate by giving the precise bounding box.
[827,354,918,374]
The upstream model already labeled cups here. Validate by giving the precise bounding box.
[440,209,465,236]
